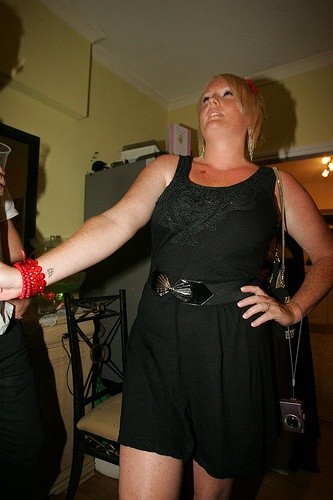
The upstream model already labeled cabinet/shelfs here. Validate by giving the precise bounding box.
[270,156,333,335]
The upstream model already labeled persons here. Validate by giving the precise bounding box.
[0,169,69,500]
[0,74,333,500]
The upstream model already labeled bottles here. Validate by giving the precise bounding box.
[34,235,66,314]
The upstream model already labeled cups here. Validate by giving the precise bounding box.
[0,143,12,170]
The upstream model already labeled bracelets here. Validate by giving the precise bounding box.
[13,258,45,299]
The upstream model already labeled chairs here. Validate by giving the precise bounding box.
[62,290,129,500]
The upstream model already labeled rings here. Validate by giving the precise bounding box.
[265,302,270,311]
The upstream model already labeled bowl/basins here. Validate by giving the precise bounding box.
[46,271,87,293]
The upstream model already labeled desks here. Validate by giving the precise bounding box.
[39,312,96,497]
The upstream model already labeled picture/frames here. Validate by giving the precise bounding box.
[0,121,40,260]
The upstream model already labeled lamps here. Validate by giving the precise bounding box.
[321,154,333,177]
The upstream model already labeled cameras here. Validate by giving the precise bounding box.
[281,398,305,433]
